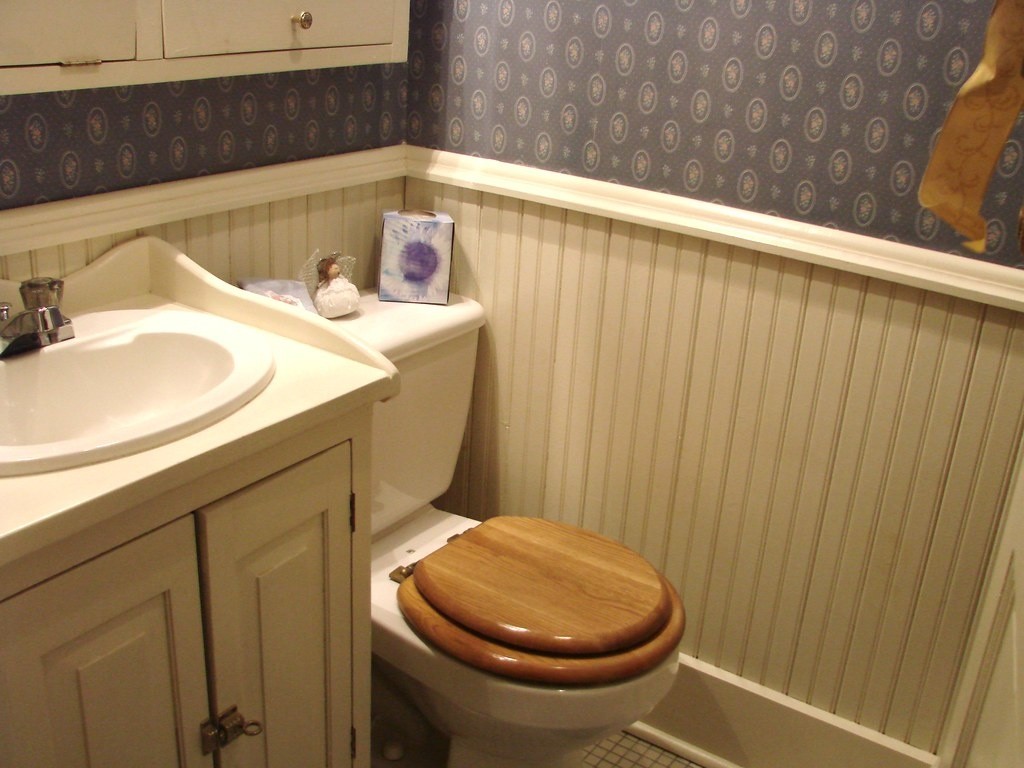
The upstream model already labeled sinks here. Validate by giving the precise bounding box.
[0,310,278,476]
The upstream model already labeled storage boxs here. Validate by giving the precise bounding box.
[376,207,457,304]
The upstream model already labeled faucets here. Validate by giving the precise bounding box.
[0,306,65,357]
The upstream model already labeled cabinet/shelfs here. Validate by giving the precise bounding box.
[0,397,376,768]
[0,0,410,97]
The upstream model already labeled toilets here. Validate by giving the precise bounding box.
[313,285,685,768]
